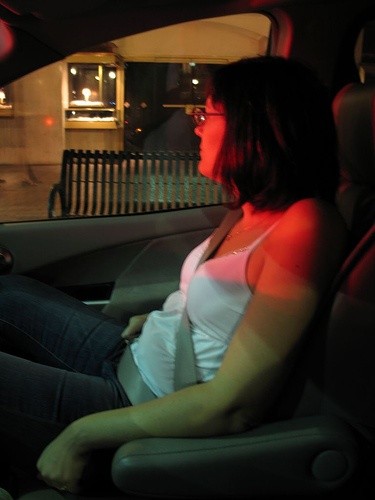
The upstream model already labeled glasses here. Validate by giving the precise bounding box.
[191,107,226,127]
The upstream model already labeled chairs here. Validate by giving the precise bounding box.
[111,83,375,500]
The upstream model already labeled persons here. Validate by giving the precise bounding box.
[0,54,346,499]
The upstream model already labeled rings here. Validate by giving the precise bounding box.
[56,484,66,493]
